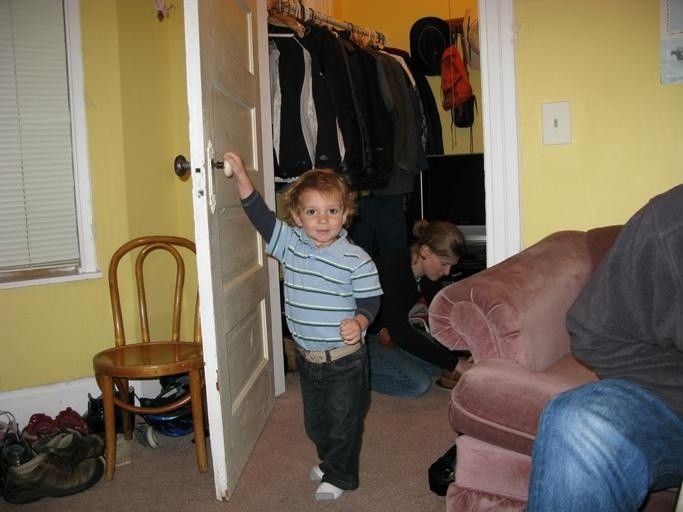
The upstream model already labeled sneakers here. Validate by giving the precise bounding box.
[135,420,160,449]
[0,387,136,504]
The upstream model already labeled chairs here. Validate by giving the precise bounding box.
[425,224,681,511]
[92,235,209,481]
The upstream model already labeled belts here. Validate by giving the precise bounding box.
[294,338,365,364]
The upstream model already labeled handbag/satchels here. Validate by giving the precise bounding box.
[441,46,472,111]
[451,96,475,128]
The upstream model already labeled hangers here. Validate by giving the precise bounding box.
[267,0,408,60]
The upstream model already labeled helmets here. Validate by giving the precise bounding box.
[141,373,194,437]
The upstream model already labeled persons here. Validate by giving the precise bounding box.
[365,217,476,397]
[222,150,385,502]
[526,183,683,511]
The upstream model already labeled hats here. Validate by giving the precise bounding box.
[410,17,455,76]
[463,8,480,71]
